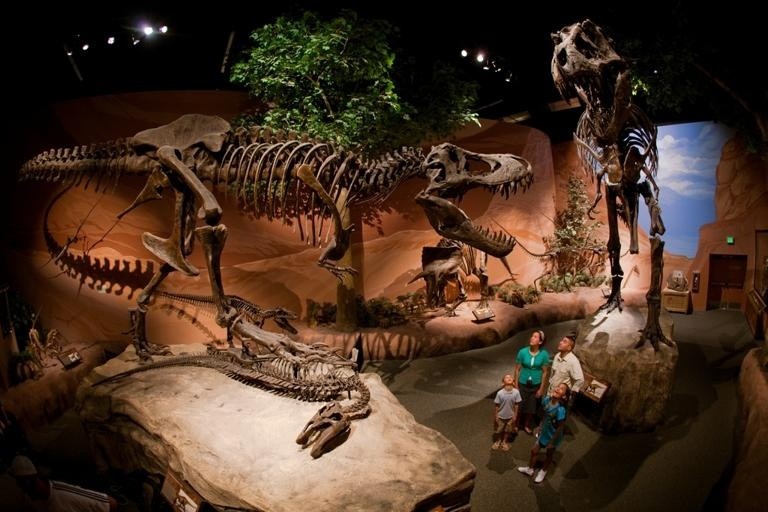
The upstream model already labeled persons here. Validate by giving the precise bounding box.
[514,330,550,435]
[536,335,585,436]
[491,375,523,451]
[518,384,570,483]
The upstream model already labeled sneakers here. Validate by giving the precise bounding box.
[517,466,534,476]
[491,424,540,451]
[534,468,548,482]
